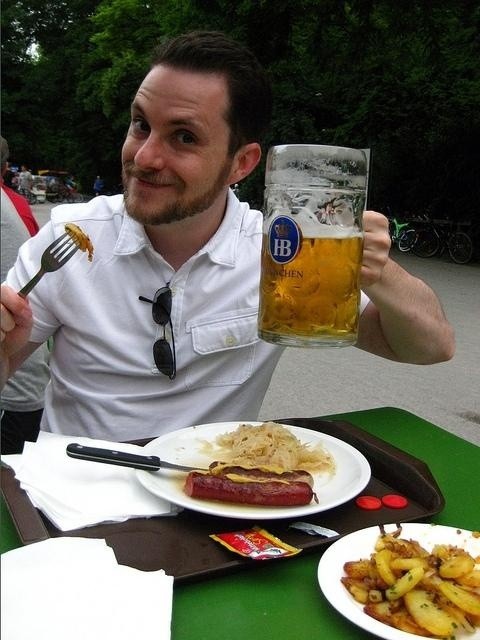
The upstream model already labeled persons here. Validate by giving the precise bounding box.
[0,136,50,456]
[12,164,32,196]
[0,31,457,452]
[4,162,16,191]
[93,175,104,192]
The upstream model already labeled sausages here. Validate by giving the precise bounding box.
[185,471,313,506]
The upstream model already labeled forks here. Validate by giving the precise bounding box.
[18,229,83,297]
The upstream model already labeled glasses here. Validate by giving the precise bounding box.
[137,282,176,381]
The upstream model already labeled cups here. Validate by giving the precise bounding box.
[253,143,369,349]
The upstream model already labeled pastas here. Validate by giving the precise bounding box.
[217,420,335,471]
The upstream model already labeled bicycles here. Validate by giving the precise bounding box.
[381,203,476,267]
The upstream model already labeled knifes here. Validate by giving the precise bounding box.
[67,440,214,473]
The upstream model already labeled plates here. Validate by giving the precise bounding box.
[318,522,479,640]
[137,418,370,518]
